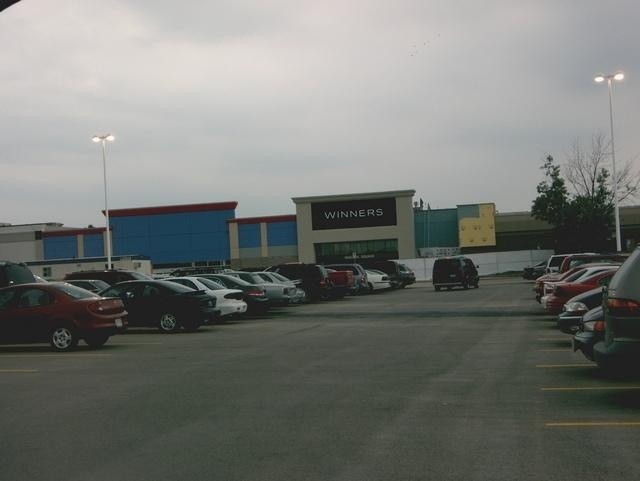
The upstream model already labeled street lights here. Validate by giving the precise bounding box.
[592,70,627,254]
[91,134,117,273]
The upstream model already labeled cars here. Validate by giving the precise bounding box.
[62,258,416,323]
[0,259,37,289]
[97,279,221,334]
[522,242,640,385]
[0,281,130,353]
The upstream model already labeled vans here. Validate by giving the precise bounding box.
[431,257,483,294]
